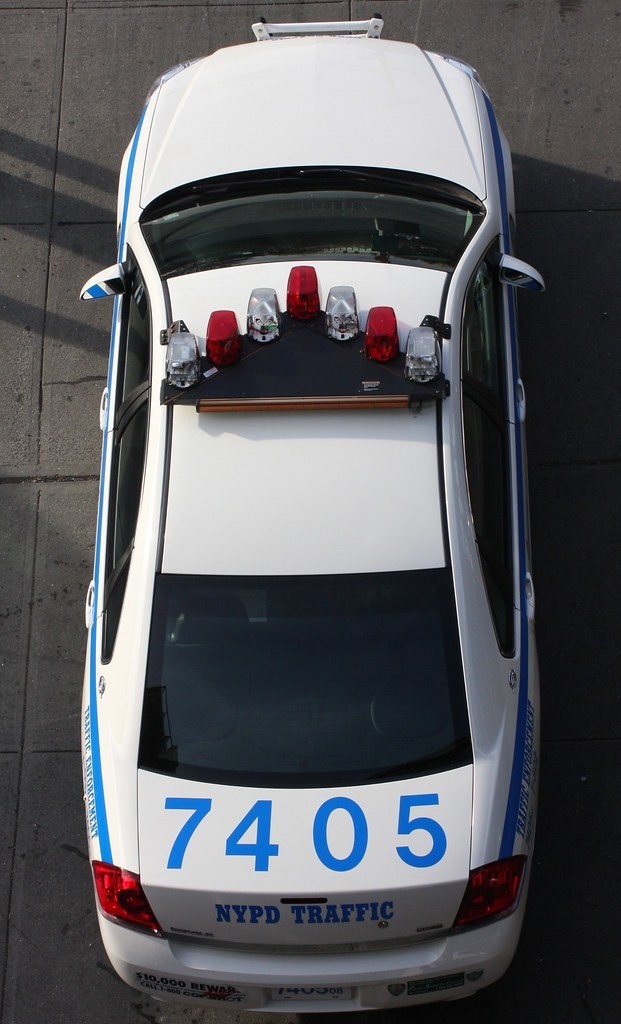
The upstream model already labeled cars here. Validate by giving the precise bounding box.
[74,12,547,1015]
